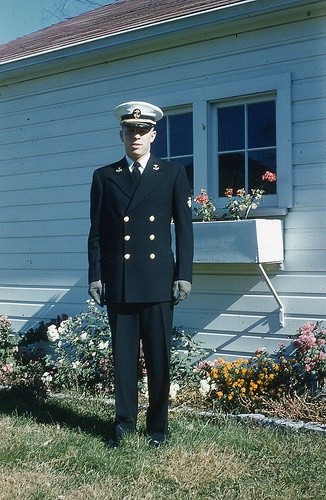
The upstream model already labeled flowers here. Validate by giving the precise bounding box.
[193,165,284,222]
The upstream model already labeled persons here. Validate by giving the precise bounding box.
[88,101,194,446]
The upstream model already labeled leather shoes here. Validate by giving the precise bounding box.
[149,438,160,447]
[108,439,119,450]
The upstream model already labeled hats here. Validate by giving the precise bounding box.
[113,100,164,129]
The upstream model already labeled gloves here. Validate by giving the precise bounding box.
[88,280,105,308]
[172,279,191,304]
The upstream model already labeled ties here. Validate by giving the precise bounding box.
[131,161,143,186]
